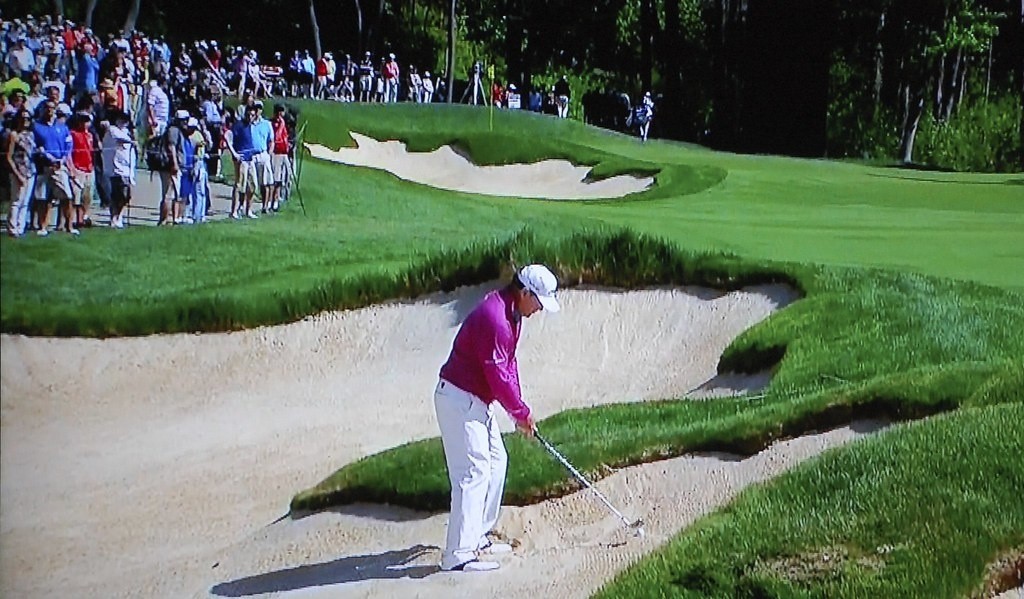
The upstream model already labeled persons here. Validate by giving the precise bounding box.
[0,11,654,237]
[435,265,561,571]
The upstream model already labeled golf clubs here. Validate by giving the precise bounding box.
[532,429,644,529]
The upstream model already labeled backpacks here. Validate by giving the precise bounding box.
[147,130,172,171]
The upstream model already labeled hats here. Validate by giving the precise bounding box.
[0,13,431,78]
[80,112,93,122]
[100,79,115,89]
[518,264,561,312]
[252,100,264,110]
[56,105,72,113]
[175,110,190,120]
[274,103,285,113]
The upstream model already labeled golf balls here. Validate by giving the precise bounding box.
[637,529,646,537]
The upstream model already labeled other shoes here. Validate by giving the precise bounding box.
[78,217,92,229]
[454,560,499,572]
[37,230,47,235]
[230,213,241,220]
[247,212,257,219]
[11,232,22,240]
[110,219,124,229]
[69,228,79,235]
[476,539,512,557]
